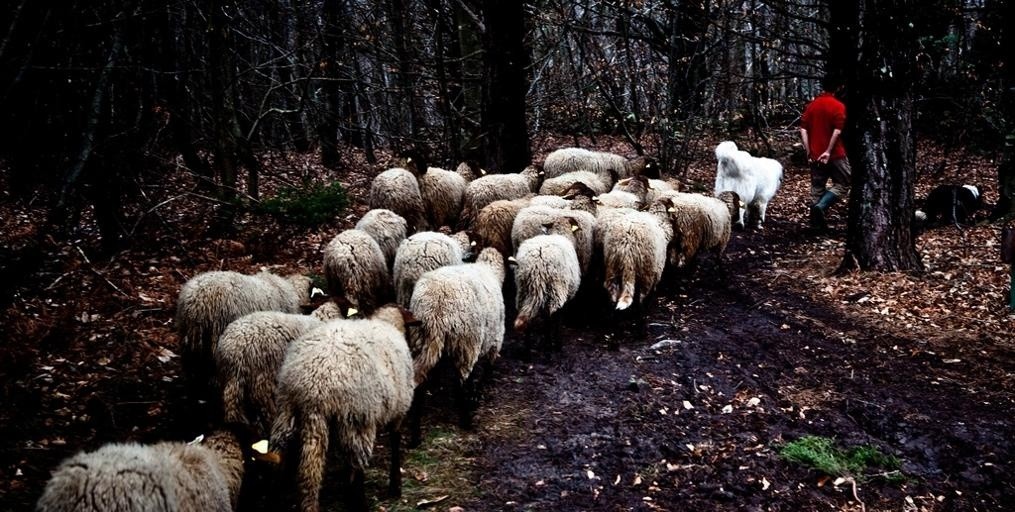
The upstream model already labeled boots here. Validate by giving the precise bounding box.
[814,191,838,225]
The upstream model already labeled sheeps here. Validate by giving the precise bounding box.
[326,229,389,317]
[355,208,408,271]
[370,164,424,229]
[423,161,474,230]
[590,201,675,309]
[396,228,469,309]
[465,160,543,231]
[258,302,416,511]
[654,191,733,284]
[543,146,641,180]
[512,205,597,256]
[35,425,246,511]
[176,269,316,374]
[648,177,679,210]
[539,171,615,198]
[508,235,582,326]
[217,308,353,431]
[595,177,647,217]
[411,245,509,389]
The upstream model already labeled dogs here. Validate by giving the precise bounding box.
[713,140,785,231]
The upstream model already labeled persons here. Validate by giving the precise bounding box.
[799,74,852,229]
